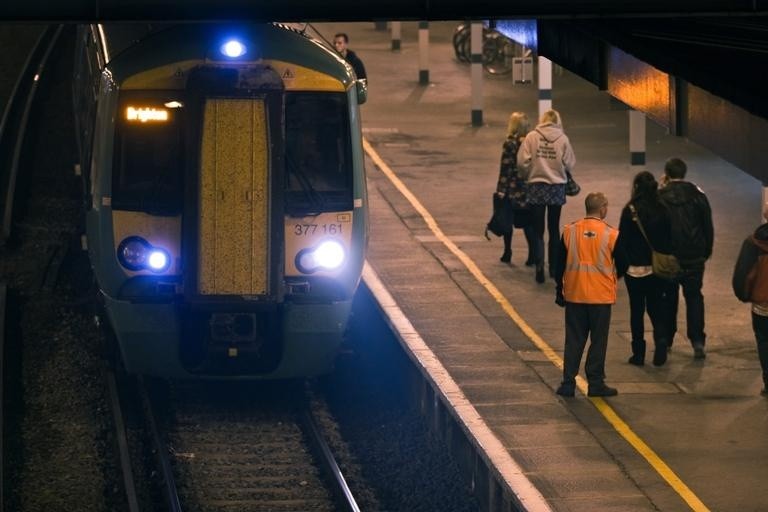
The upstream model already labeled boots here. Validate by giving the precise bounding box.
[629,340,646,365]
[534,239,545,282]
[653,324,669,366]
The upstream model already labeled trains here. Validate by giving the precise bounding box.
[68,19,372,405]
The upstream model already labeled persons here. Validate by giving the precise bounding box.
[730,201,768,398]
[515,108,576,284]
[547,191,632,398]
[493,111,542,266]
[332,33,368,90]
[652,157,715,360]
[615,170,675,366]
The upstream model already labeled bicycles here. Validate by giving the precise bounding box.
[453,21,565,83]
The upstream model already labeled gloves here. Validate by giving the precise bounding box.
[555,286,564,307]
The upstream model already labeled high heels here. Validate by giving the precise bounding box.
[501,248,512,263]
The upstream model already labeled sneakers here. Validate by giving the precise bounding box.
[693,340,706,360]
[588,381,617,396]
[557,382,575,396]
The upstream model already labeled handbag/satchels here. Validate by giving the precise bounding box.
[566,170,580,196]
[486,193,513,236]
[651,251,680,279]
